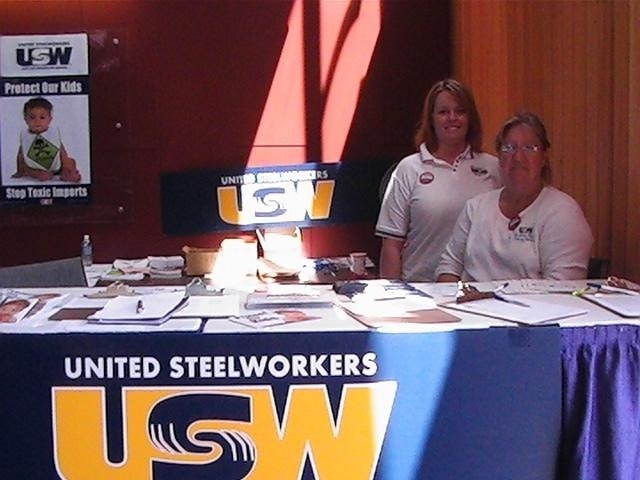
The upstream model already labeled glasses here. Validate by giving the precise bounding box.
[501,143,546,153]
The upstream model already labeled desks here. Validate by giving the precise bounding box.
[3,276,639,480]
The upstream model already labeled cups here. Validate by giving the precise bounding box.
[351,253,367,276]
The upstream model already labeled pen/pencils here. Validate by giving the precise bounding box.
[137,301,143,313]
[586,282,640,296]
[457,277,463,291]
[494,283,509,293]
[582,286,590,291]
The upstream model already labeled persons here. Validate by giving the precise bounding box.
[434,112,595,282]
[0,300,29,323]
[13,98,81,182]
[373,79,502,282]
[276,310,318,324]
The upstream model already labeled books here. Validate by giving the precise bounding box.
[88,296,191,325]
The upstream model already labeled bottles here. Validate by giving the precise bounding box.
[81,234,92,267]
